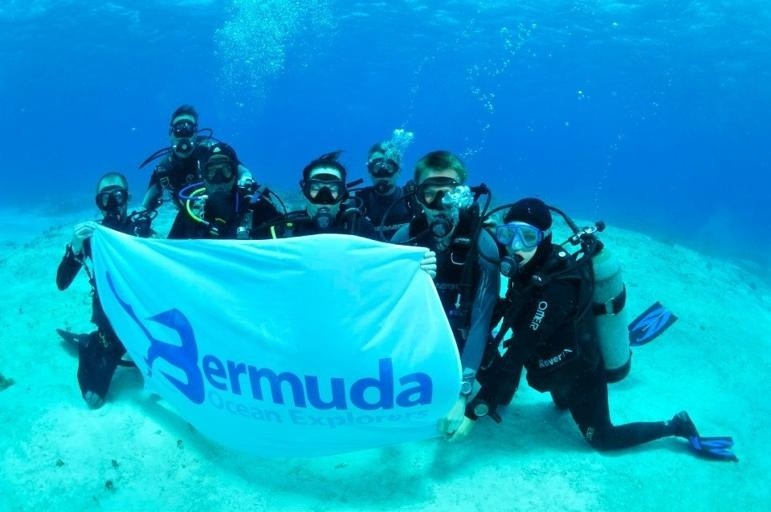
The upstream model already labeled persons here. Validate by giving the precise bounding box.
[141,105,253,211]
[57,173,157,408]
[257,151,378,239]
[447,198,738,461]
[167,142,284,239]
[391,150,524,433]
[343,141,421,243]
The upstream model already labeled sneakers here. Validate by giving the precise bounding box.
[77,333,90,350]
[671,410,701,445]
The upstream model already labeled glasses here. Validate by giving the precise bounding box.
[172,120,195,138]
[415,177,462,210]
[203,160,235,183]
[303,177,346,205]
[367,158,398,178]
[96,188,128,210]
[494,224,551,249]
